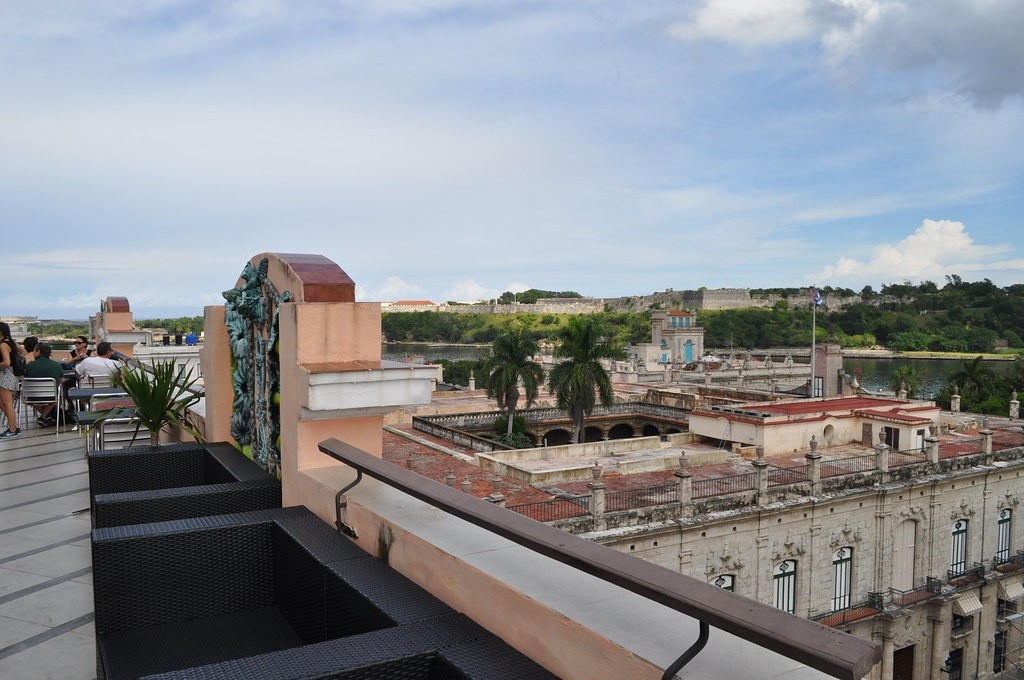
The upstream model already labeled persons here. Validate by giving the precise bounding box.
[0,322,27,438]
[63,336,125,430]
[20,337,63,427]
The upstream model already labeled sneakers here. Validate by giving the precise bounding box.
[0,429,19,439]
[4,428,22,435]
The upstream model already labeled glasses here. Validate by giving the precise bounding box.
[73,341,84,345]
[109,349,114,352]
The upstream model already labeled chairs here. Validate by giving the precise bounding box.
[0,374,151,450]
[85,440,561,680]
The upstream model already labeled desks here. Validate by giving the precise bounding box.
[66,387,131,434]
[75,409,140,450]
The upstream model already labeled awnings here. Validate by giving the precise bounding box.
[953,590,983,616]
[997,576,1024,602]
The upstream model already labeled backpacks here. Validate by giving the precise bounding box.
[4,340,26,377]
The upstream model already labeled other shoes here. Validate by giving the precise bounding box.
[36,417,49,426]
[72,425,78,430]
[46,417,56,424]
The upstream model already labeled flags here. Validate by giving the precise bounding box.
[813,287,822,307]
